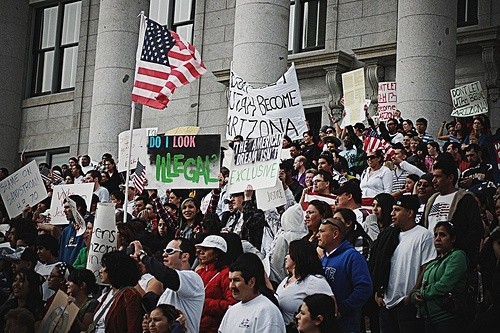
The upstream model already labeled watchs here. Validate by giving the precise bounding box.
[137,249,147,261]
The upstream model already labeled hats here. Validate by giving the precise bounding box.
[320,125,328,134]
[323,136,341,147]
[0,246,14,260]
[353,123,365,129]
[407,174,420,183]
[418,173,434,190]
[332,183,362,197]
[393,193,420,210]
[446,121,456,129]
[5,246,38,262]
[68,157,78,163]
[195,235,228,254]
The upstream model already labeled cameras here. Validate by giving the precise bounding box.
[127,245,134,255]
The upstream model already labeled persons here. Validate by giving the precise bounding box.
[127,237,205,333]
[0,117,500,333]
[218,258,287,333]
[4,308,36,333]
[409,221,477,333]
[368,196,437,333]
[66,268,99,333]
[194,235,237,333]
[296,293,336,333]
[93,250,143,333]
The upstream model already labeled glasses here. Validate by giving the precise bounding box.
[103,157,106,160]
[366,155,379,160]
[321,219,342,232]
[387,123,395,127]
[50,275,62,278]
[104,164,110,165]
[163,247,183,254]
[325,130,332,134]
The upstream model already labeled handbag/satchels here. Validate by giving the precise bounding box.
[80,287,124,333]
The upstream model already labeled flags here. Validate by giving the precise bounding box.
[362,128,395,162]
[132,16,207,109]
